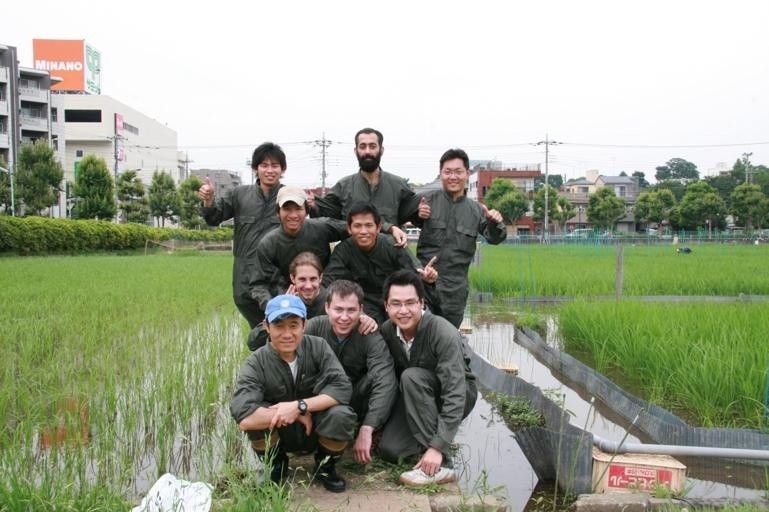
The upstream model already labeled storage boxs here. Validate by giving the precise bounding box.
[591,443,688,500]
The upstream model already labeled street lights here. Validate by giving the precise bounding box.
[578,205,584,243]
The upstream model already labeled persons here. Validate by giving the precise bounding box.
[248,186,408,312]
[372,269,478,487]
[247,251,378,351]
[304,279,399,465]
[229,294,359,493]
[197,142,341,329]
[304,128,431,226]
[398,148,507,330]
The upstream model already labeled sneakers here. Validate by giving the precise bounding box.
[400,459,458,488]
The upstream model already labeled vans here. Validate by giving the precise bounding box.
[566,228,594,238]
[405,228,421,239]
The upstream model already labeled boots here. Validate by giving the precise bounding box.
[251,434,289,488]
[311,435,347,492]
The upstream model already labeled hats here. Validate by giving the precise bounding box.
[264,295,307,324]
[276,184,307,210]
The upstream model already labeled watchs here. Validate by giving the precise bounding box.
[298,398,308,415]
